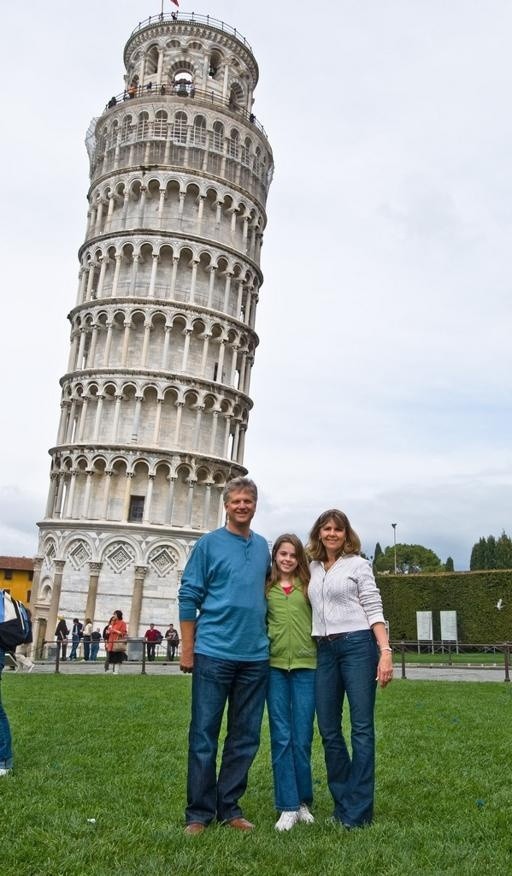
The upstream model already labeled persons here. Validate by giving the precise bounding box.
[14,608,34,673]
[306,509,393,830]
[54,610,126,675]
[265,534,317,832]
[144,623,160,661]
[175,476,271,837]
[165,624,178,661]
[0,591,14,778]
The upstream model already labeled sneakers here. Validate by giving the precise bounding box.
[274,811,299,833]
[299,803,314,825]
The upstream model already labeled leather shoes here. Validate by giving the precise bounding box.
[229,818,255,831]
[183,823,206,836]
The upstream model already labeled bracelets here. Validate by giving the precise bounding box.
[379,647,393,652]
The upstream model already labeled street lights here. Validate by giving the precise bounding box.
[390,521,399,577]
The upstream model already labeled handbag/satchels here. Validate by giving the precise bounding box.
[3,590,33,647]
[112,640,128,651]
